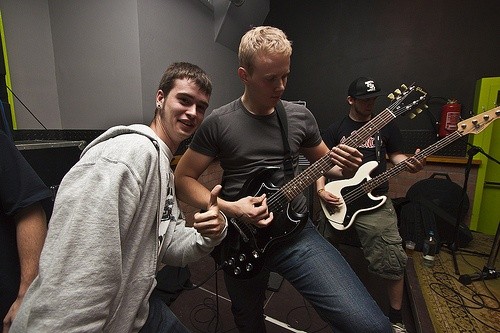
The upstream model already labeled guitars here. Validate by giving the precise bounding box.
[207,83,430,278]
[318,102,500,230]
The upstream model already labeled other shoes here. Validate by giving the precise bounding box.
[391,322,408,333]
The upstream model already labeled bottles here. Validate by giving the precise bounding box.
[422,231,438,271]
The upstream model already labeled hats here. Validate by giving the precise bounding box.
[346,75,386,100]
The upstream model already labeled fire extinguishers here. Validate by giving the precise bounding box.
[439,98,463,138]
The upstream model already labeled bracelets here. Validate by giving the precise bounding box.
[318,189,324,194]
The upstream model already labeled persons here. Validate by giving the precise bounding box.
[0,130,52,333]
[174,26,393,332]
[317,76,427,333]
[9,61,228,333]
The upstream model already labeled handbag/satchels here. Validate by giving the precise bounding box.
[406,171,469,222]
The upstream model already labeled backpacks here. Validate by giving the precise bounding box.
[399,199,437,251]
